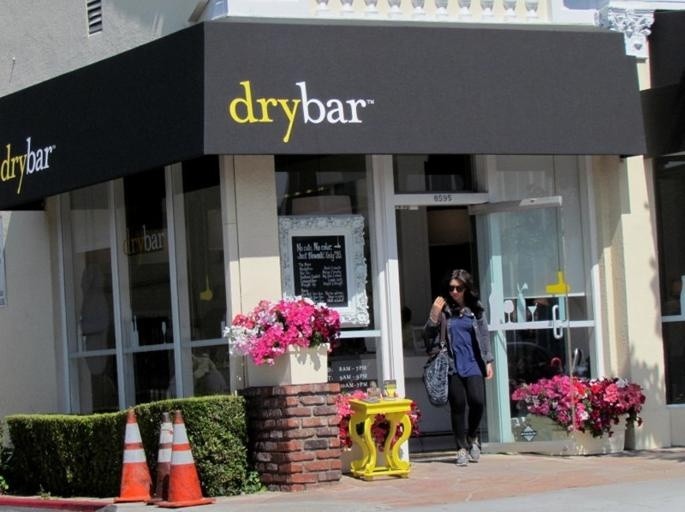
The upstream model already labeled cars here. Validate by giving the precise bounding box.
[505,341,567,394]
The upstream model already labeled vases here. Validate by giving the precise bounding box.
[524,412,630,456]
[340,435,409,474]
[247,343,328,388]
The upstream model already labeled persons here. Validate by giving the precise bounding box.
[421,268,493,466]
[400,306,420,348]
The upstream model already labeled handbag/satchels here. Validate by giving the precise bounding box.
[423,348,449,408]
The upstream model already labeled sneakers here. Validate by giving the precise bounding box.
[471,445,480,462]
[456,449,468,466]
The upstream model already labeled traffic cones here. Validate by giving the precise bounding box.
[113,405,219,507]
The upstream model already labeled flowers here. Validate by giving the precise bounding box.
[223,292,341,367]
[512,376,646,438]
[338,389,422,447]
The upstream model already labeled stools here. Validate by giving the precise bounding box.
[348,399,414,480]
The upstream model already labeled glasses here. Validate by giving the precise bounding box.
[449,284,463,293]
